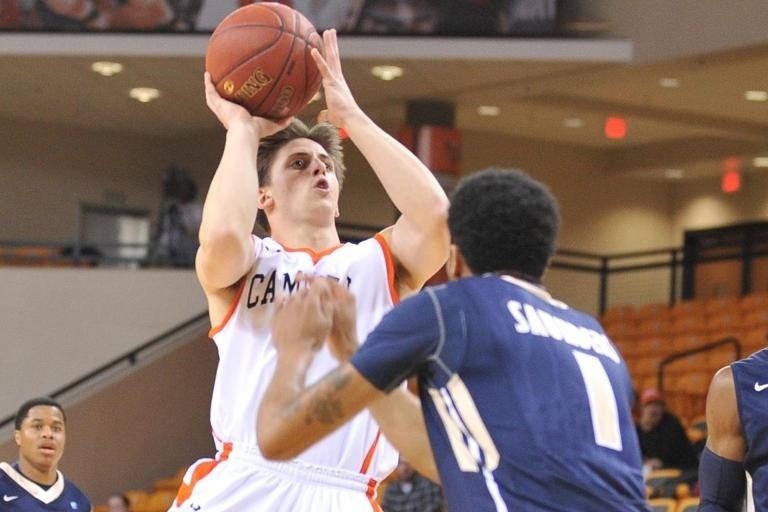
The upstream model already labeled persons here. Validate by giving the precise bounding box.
[637,389,695,468]
[107,493,129,512]
[255,168,649,512]
[0,397,92,512]
[380,460,442,511]
[693,344,768,510]
[162,27,451,512]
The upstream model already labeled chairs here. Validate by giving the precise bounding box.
[596,289,768,510]
[89,466,188,511]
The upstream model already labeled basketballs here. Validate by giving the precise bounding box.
[206,1,325,122]
